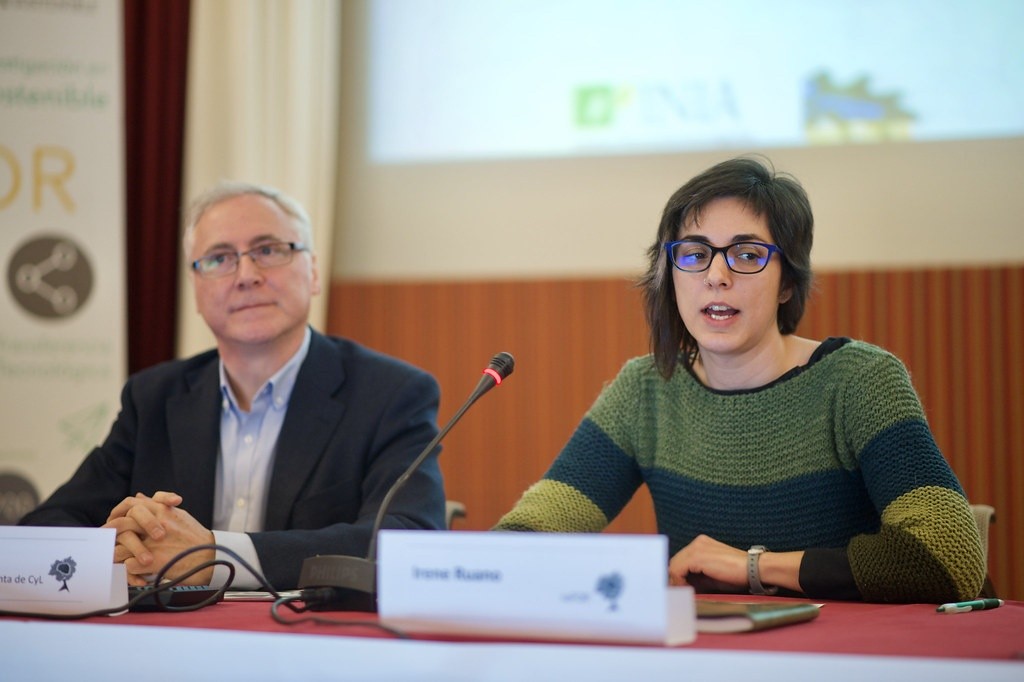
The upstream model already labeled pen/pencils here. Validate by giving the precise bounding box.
[935,599,1004,614]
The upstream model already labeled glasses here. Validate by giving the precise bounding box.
[664,239,782,274]
[191,241,310,279]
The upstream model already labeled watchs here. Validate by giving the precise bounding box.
[747,545,779,595]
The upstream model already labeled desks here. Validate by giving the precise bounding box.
[0,590,1024,682]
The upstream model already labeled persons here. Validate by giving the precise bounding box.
[17,182,446,592]
[488,159,986,604]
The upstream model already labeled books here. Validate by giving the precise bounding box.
[695,598,819,634]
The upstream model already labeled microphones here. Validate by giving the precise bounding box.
[299,351,515,612]
